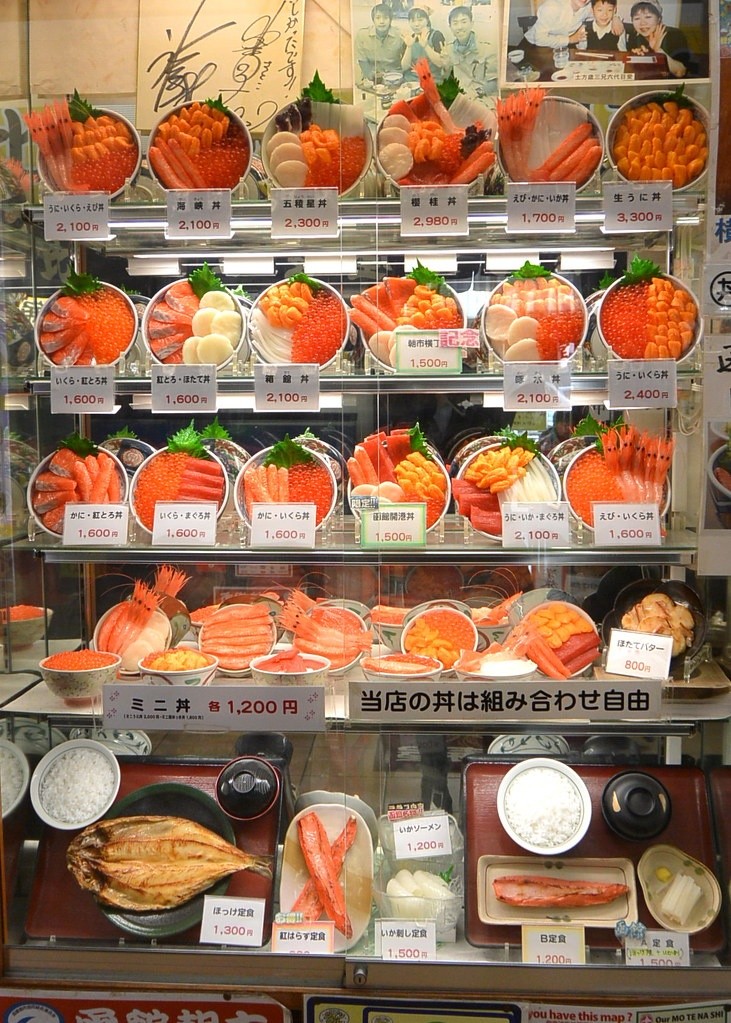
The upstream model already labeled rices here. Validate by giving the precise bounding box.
[38,750,114,825]
[505,768,582,848]
[0,745,23,815]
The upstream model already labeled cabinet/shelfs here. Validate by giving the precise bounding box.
[0,194,731,1013]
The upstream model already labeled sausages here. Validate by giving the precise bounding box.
[148,123,604,191]
[73,447,378,521]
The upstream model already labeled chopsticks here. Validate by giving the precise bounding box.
[575,52,614,59]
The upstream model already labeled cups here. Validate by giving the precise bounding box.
[553,47,569,68]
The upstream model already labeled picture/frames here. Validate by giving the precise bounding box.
[497,0,711,89]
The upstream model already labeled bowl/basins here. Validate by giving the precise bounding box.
[361,654,538,684]
[400,82,420,96]
[602,772,672,842]
[1,606,53,648]
[379,809,466,874]
[214,755,279,824]
[382,72,403,87]
[0,717,150,756]
[508,49,524,63]
[472,615,511,651]
[38,650,122,702]
[237,732,293,767]
[138,652,218,691]
[497,758,593,858]
[489,735,571,762]
[375,858,466,940]
[249,653,331,688]
[551,70,573,82]
[372,617,408,651]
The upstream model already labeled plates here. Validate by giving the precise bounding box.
[90,780,237,938]
[141,278,246,374]
[147,100,253,195]
[281,803,374,953]
[93,602,597,679]
[375,95,496,192]
[34,281,140,370]
[26,437,674,540]
[606,90,711,192]
[480,270,589,364]
[249,276,352,373]
[597,271,704,364]
[295,790,379,851]
[476,843,721,936]
[359,276,468,372]
[603,578,706,669]
[0,738,121,831]
[498,96,605,193]
[36,108,142,202]
[261,99,373,199]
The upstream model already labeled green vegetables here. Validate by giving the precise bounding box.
[59,249,696,470]
[67,68,693,123]
